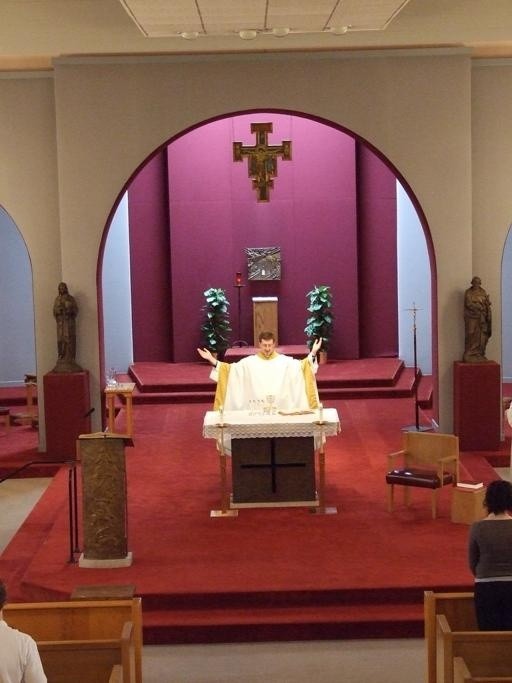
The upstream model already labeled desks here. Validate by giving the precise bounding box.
[105,381,137,436]
[200,410,343,517]
[251,295,280,346]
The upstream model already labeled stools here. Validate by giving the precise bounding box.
[451,484,488,525]
[0,406,9,433]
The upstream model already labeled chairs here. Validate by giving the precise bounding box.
[386,430,463,520]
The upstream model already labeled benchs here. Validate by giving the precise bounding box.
[422,591,512,682]
[2,596,143,682]
[35,622,134,682]
[438,618,512,682]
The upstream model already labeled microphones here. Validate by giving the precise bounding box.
[76,408,95,440]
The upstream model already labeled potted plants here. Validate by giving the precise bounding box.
[303,282,334,365]
[199,287,232,364]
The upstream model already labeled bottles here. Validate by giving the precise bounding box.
[109,367,117,389]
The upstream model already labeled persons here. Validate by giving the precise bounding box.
[52,281,83,372]
[467,479,511,632]
[462,277,493,364]
[1,581,47,683]
[196,331,327,456]
[242,147,284,181]
